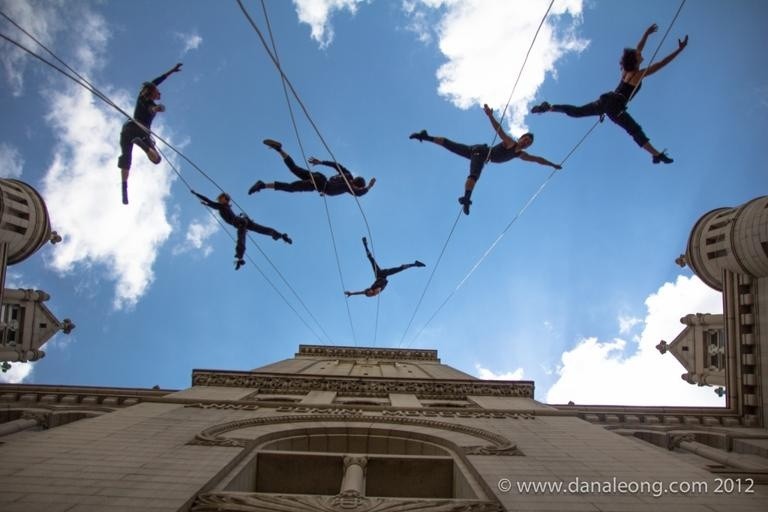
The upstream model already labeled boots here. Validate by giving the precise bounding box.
[130,136,149,151]
[122,181,128,204]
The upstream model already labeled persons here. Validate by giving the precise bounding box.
[406,102,564,218]
[245,137,377,197]
[115,61,186,205]
[343,236,429,298]
[530,21,690,165]
[189,188,292,272]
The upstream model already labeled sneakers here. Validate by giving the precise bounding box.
[262,139,282,151]
[409,129,429,142]
[531,101,550,114]
[361,237,367,246]
[282,233,292,244]
[458,196,472,215]
[235,260,246,270]
[652,152,674,164]
[415,260,425,269]
[248,180,265,195]
